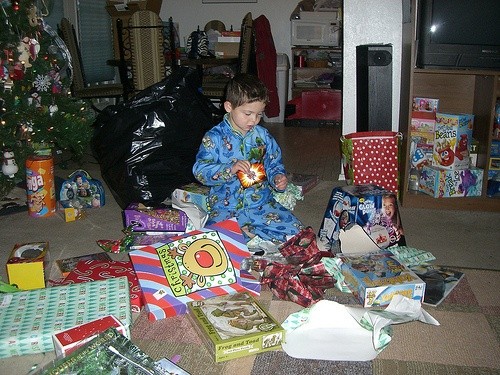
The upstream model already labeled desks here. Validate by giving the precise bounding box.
[106,57,241,103]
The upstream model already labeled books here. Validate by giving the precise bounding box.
[411,262,465,308]
[330,51,342,68]
[293,75,334,89]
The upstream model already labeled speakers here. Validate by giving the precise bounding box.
[355,44,392,132]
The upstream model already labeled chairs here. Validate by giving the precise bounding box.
[117,10,178,104]
[198,13,253,103]
[57,17,124,114]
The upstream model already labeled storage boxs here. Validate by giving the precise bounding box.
[0,276,133,358]
[128,217,261,320]
[172,183,211,213]
[215,31,240,59]
[336,250,426,308]
[292,173,320,194]
[124,202,188,232]
[62,260,144,312]
[488,104,500,197]
[186,291,285,363]
[52,314,127,359]
[409,97,484,199]
[6,241,52,291]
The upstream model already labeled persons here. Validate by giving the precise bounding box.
[193,73,306,244]
[380,193,404,237]
[338,209,350,230]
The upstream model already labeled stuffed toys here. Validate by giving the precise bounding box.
[2,147,18,174]
[18,34,40,67]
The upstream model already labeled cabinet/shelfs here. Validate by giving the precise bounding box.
[402,0,500,213]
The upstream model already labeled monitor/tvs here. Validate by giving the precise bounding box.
[419,0,500,71]
